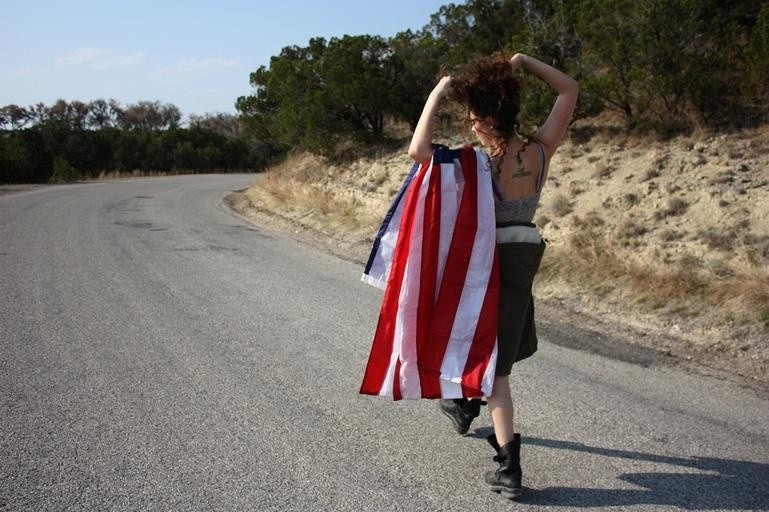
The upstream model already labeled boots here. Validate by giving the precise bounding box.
[440,397,487,435]
[483,432,522,498]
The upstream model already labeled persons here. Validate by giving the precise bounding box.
[407,52,580,499]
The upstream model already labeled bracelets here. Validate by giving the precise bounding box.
[524,54,528,69]
